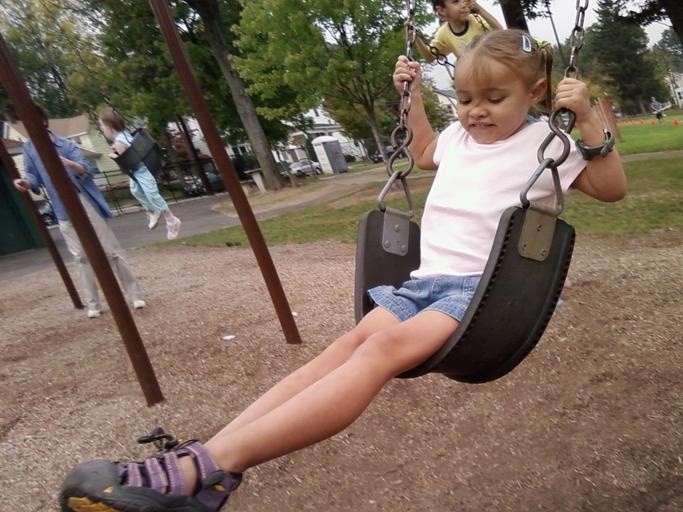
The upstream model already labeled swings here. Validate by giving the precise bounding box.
[354,1,590,384]
[18,2,165,182]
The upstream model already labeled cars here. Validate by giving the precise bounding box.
[285,159,321,177]
[370,145,404,163]
[189,172,224,194]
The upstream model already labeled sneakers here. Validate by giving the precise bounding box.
[87,308,101,318]
[132,299,145,309]
[144,209,161,230]
[165,216,181,240]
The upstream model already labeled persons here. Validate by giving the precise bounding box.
[650,96,665,123]
[13,104,147,318]
[60,28,627,512]
[406,0,503,64]
[98,108,181,240]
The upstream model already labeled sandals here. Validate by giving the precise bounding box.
[57,426,243,510]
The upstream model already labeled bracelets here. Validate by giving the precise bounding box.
[574,128,616,161]
